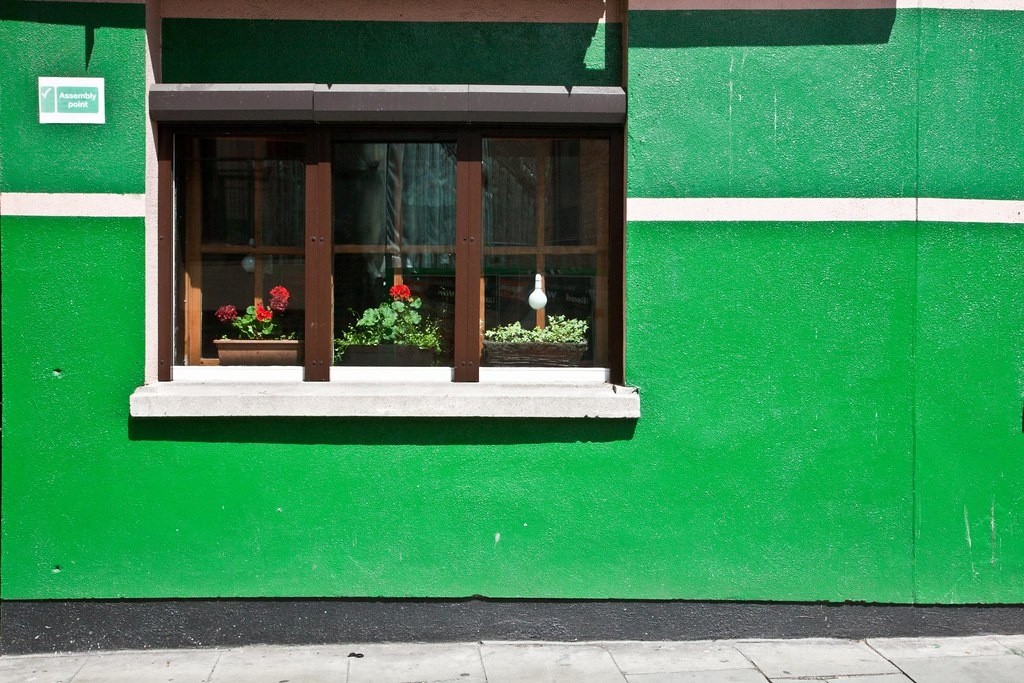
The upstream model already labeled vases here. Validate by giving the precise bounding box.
[213,340,303,366]
[341,344,450,367]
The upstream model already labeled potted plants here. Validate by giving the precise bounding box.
[482,314,590,367]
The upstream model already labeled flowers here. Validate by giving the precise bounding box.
[333,283,442,366]
[214,286,303,341]
